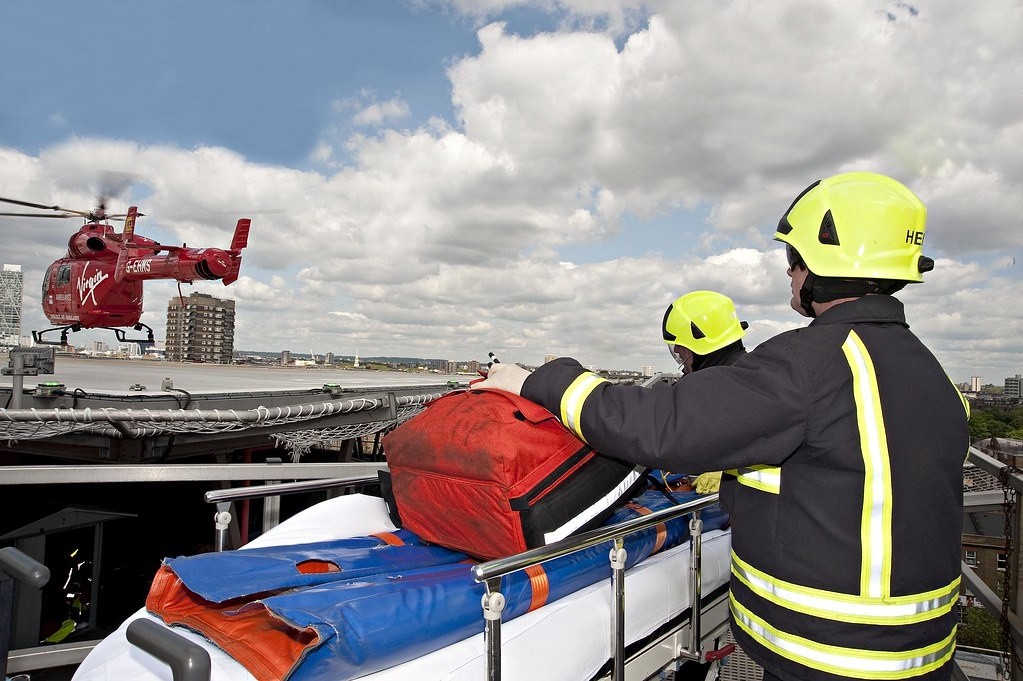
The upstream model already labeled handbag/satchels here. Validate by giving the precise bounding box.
[380,384,656,562]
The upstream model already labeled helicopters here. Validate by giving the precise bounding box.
[0,188,252,357]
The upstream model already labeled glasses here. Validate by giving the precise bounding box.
[785,242,806,272]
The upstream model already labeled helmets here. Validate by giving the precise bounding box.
[661,290,750,356]
[771,170,935,283]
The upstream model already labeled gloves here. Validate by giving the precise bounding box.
[691,470,724,495]
[470,363,533,396]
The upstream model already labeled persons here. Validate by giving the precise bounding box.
[662,290,750,494]
[472,172,971,681]
